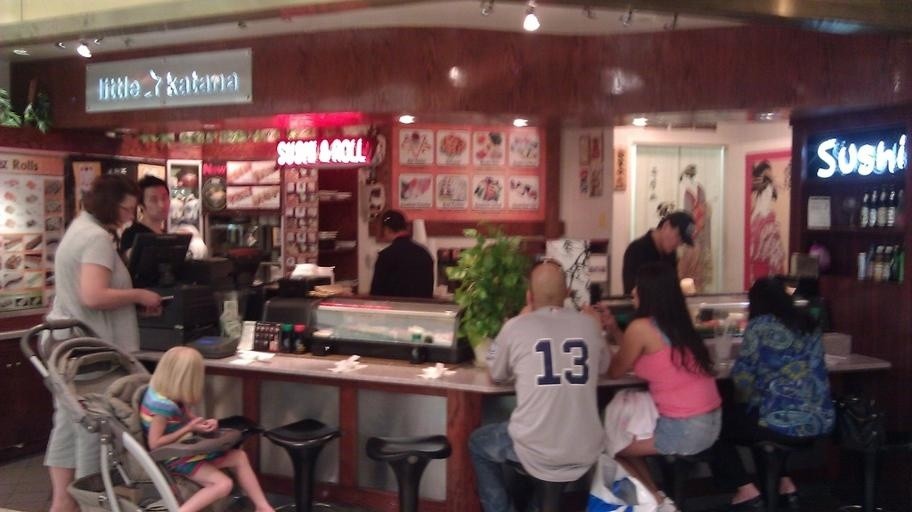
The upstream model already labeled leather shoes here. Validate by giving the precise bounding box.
[711,491,802,512]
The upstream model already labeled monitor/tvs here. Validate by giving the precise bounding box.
[128,232,192,286]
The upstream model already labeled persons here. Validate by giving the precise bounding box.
[622,211,697,295]
[730,275,839,506]
[139,347,277,511]
[603,259,724,512]
[465,257,612,511]
[177,224,208,261]
[41,173,162,511]
[369,208,434,298]
[118,174,171,262]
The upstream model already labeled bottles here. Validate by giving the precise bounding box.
[409,331,433,364]
[294,324,305,353]
[282,322,295,353]
[858,242,906,284]
[860,188,905,228]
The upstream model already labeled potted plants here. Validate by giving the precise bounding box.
[451,229,535,371]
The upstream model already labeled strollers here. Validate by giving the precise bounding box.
[14,315,269,512]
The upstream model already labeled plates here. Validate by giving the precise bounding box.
[201,175,227,211]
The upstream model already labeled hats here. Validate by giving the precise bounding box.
[380,209,407,229]
[669,210,702,248]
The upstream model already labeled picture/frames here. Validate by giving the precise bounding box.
[742,147,794,292]
[629,139,728,294]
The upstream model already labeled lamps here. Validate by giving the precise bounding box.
[478,0,683,36]
[51,32,112,60]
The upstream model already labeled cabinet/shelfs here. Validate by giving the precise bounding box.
[785,103,910,291]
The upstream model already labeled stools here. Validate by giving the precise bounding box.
[364,427,450,512]
[730,433,808,512]
[504,457,594,509]
[211,404,254,512]
[262,417,345,510]
[832,393,889,509]
[642,447,714,511]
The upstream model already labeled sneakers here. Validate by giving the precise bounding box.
[656,490,679,512]
